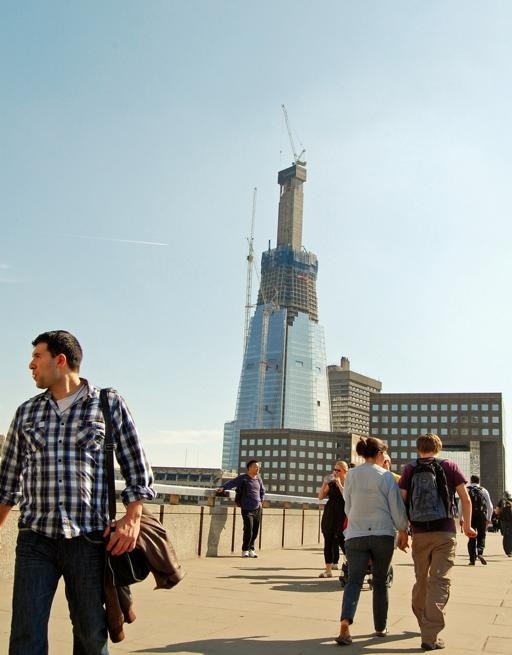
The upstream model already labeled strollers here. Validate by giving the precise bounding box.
[334,526,394,590]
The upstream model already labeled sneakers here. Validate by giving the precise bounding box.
[469,555,487,565]
[242,549,257,558]
[421,639,446,650]
[318,562,339,578]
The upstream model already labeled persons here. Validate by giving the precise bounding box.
[316,462,512,590]
[332,437,409,645]
[217,461,265,557]
[397,434,477,649]
[1,331,155,654]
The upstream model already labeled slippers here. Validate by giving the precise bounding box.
[375,628,389,637]
[335,635,352,645]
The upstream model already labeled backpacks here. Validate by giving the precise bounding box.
[409,457,454,523]
[500,498,512,522]
[466,486,487,517]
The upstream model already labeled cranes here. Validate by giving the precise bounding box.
[279,103,307,166]
[243,187,282,430]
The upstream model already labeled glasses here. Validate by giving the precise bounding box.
[333,469,341,472]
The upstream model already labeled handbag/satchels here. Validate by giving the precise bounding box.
[108,547,151,587]
